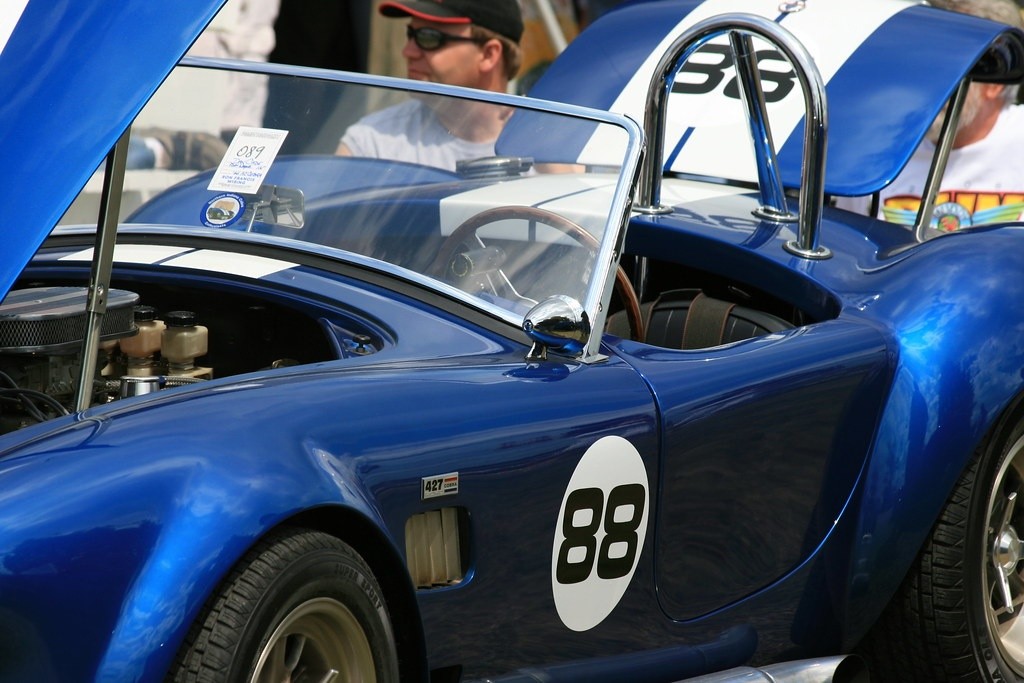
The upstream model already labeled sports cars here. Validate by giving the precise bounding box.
[0,0,1023,683]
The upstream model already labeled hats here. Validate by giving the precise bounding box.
[379,0,523,44]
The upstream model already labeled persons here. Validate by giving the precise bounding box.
[333,0,525,177]
[828,0,1024,232]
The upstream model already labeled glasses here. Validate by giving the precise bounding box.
[405,24,484,51]
